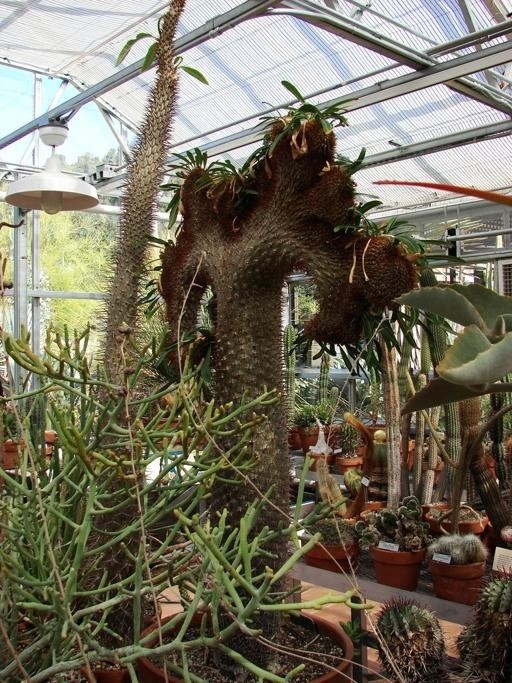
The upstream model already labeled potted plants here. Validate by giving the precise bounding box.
[1,79,512,682]
